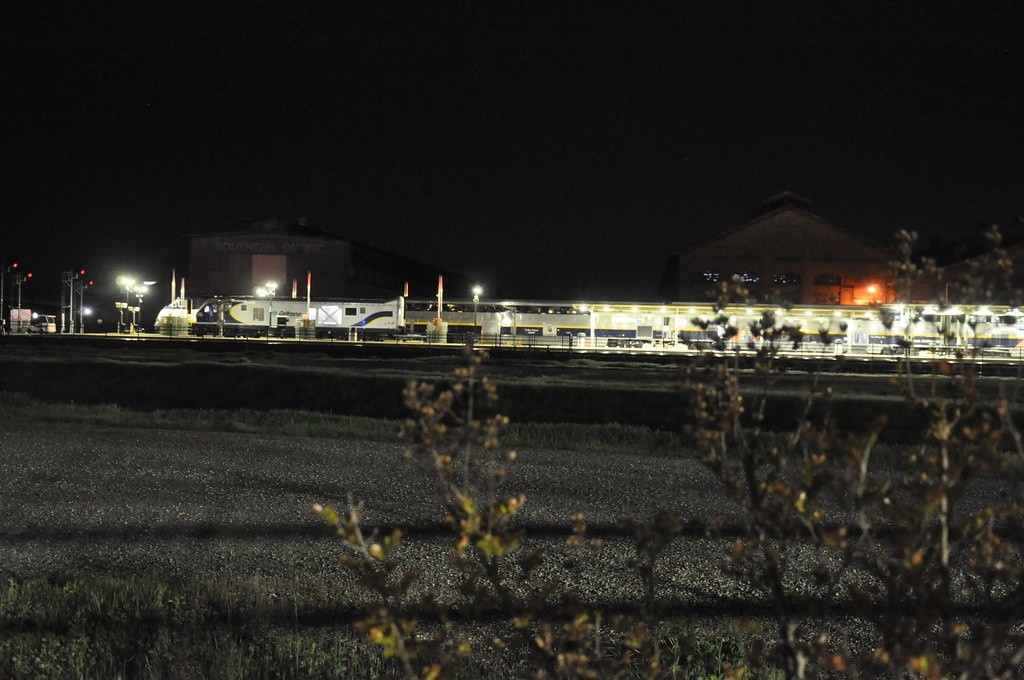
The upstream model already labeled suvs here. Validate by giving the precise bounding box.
[27,314,57,333]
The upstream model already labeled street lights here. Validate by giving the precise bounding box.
[471,283,484,335]
[121,277,134,333]
[134,285,148,332]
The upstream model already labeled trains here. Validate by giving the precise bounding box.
[153,296,199,331]
[189,297,1024,361]
